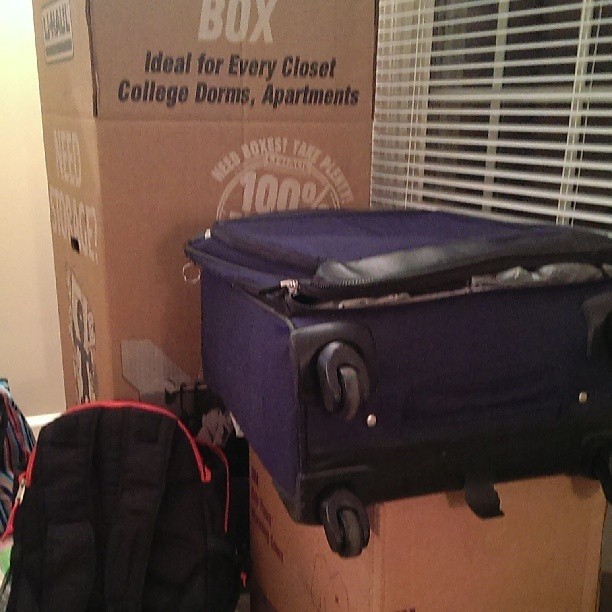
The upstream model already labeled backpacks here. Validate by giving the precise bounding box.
[0,399,247,612]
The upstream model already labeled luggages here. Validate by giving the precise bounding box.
[181,208,611,558]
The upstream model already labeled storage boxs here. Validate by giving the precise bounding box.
[34,0,378,426]
[248,441,608,612]
